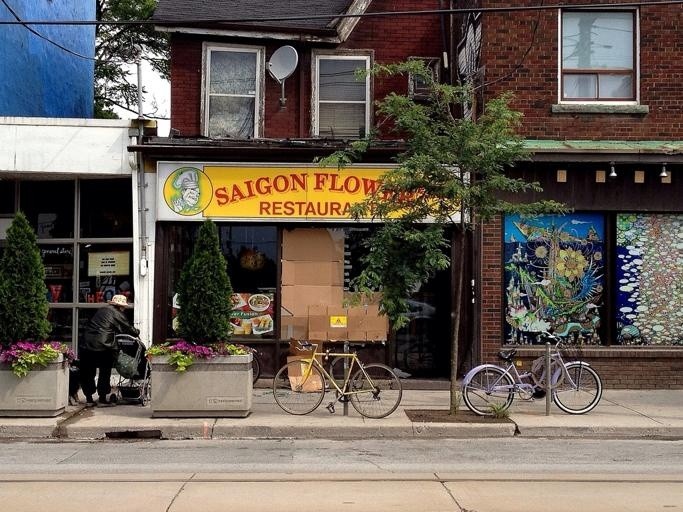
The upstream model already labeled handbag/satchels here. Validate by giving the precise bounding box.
[116,341,142,379]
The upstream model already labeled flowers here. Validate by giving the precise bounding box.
[0,340,77,378]
[144,339,249,372]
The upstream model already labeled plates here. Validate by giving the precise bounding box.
[229,292,274,334]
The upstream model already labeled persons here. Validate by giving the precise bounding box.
[79,295,140,407]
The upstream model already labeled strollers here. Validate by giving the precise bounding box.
[105,334,151,407]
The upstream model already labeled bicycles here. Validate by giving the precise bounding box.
[273,340,402,419]
[461,331,602,416]
[252,348,260,384]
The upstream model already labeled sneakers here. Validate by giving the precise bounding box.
[85,399,112,408]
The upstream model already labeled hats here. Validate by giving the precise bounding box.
[107,294,129,309]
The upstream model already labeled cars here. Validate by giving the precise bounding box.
[400,298,436,317]
